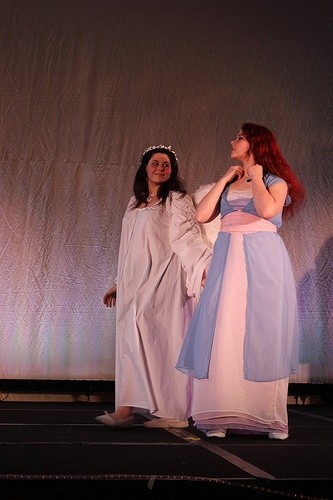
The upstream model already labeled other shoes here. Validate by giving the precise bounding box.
[96,411,189,428]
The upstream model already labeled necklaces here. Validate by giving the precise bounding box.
[243,177,252,183]
[146,193,157,202]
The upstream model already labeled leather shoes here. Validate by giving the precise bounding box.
[268,429,289,441]
[205,429,226,439]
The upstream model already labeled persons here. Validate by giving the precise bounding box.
[95,144,214,427]
[174,123,304,440]
